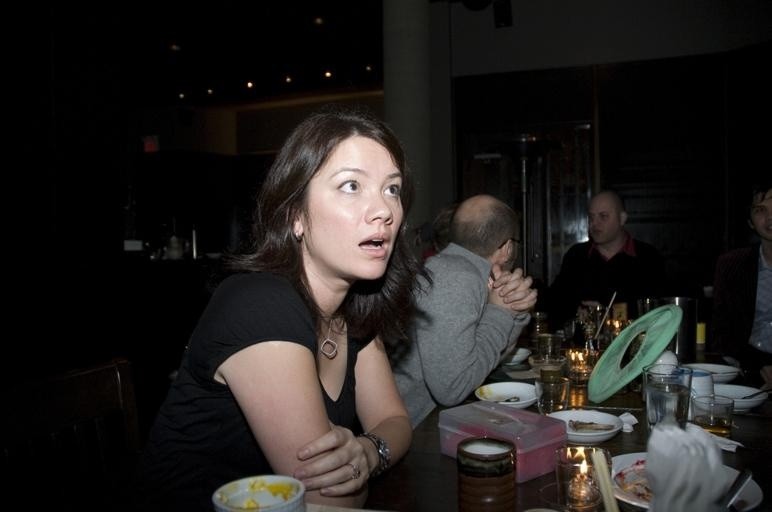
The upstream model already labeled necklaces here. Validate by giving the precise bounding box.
[312,318,342,361]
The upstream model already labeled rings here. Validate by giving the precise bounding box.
[346,462,361,480]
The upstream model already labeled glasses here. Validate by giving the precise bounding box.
[498,237,522,249]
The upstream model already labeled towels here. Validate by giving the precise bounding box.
[641,418,729,512]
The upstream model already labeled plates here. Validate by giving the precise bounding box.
[546,407,622,442]
[612,451,765,509]
[474,381,538,408]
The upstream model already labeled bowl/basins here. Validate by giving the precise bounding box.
[506,348,531,365]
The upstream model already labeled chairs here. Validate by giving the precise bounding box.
[106,340,201,510]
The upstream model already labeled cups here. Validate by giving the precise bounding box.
[534,376,569,413]
[211,474,307,511]
[537,332,561,358]
[456,435,517,509]
[643,350,768,420]
[692,394,734,438]
[552,444,613,509]
[645,364,692,432]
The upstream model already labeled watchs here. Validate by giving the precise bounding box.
[360,433,393,470]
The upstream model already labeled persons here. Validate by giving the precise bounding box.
[144,103,433,511]
[535,190,673,348]
[387,194,539,430]
[712,178,772,388]
